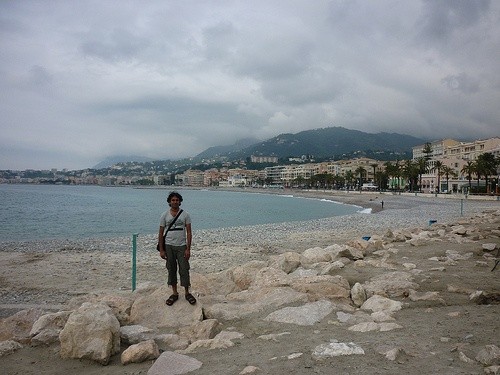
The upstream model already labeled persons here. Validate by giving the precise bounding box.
[380,200,384,208]
[156,192,197,306]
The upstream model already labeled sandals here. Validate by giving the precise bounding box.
[166,292,179,305]
[186,293,196,305]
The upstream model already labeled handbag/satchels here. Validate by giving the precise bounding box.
[156,236,166,253]
